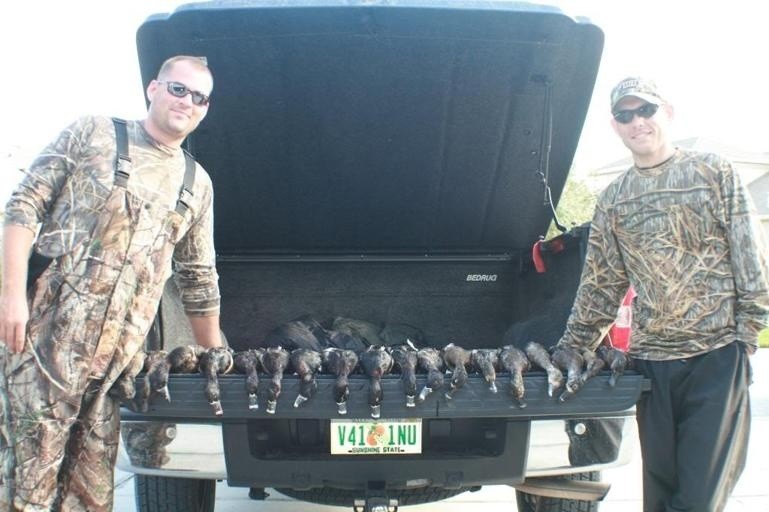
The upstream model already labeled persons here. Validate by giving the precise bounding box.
[550,74,769,512]
[0,54,233,512]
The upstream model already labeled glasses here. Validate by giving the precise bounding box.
[614,103,658,121]
[158,80,210,107]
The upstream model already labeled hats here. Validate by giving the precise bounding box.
[610,75,667,114]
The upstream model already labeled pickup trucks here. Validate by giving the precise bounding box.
[120,0,652,512]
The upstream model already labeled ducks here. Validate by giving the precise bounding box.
[440,342,635,409]
[119,346,266,417]
[259,315,445,420]
